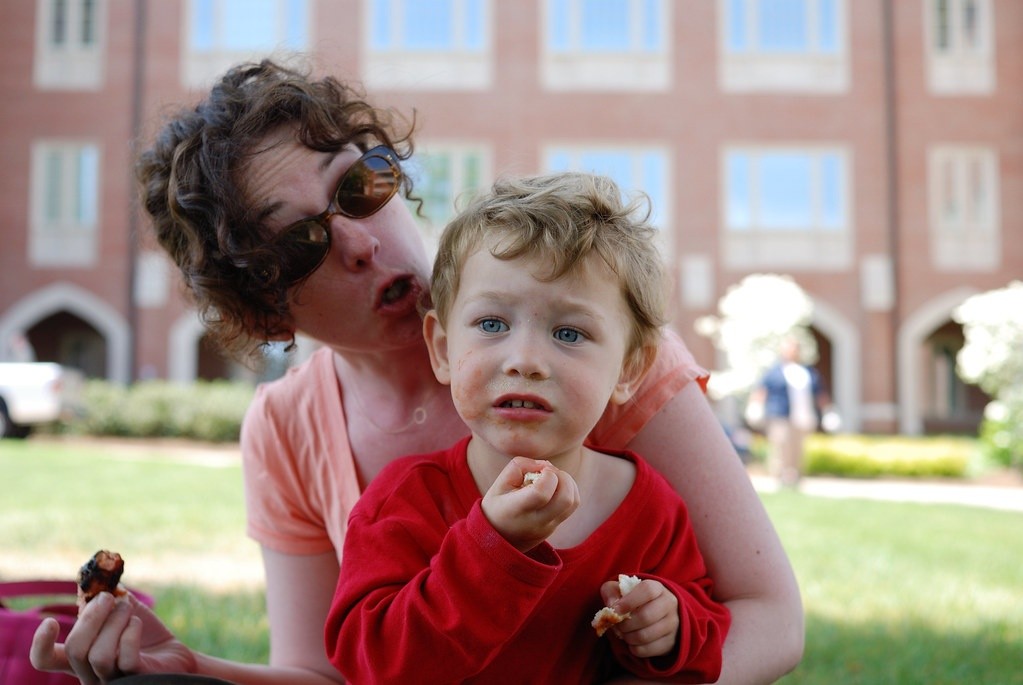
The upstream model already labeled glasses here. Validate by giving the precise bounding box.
[263,141,400,285]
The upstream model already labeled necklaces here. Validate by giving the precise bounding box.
[340,358,436,434]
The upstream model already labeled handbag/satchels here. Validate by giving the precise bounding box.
[2,581,156,685]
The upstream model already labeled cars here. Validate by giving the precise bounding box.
[0,362,61,440]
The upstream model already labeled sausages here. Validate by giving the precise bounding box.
[77,550,129,615]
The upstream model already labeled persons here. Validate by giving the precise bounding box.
[744,329,842,493]
[30,59,804,685]
[323,171,731,685]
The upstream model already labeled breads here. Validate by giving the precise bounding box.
[592,574,642,636]
[521,470,541,485]
